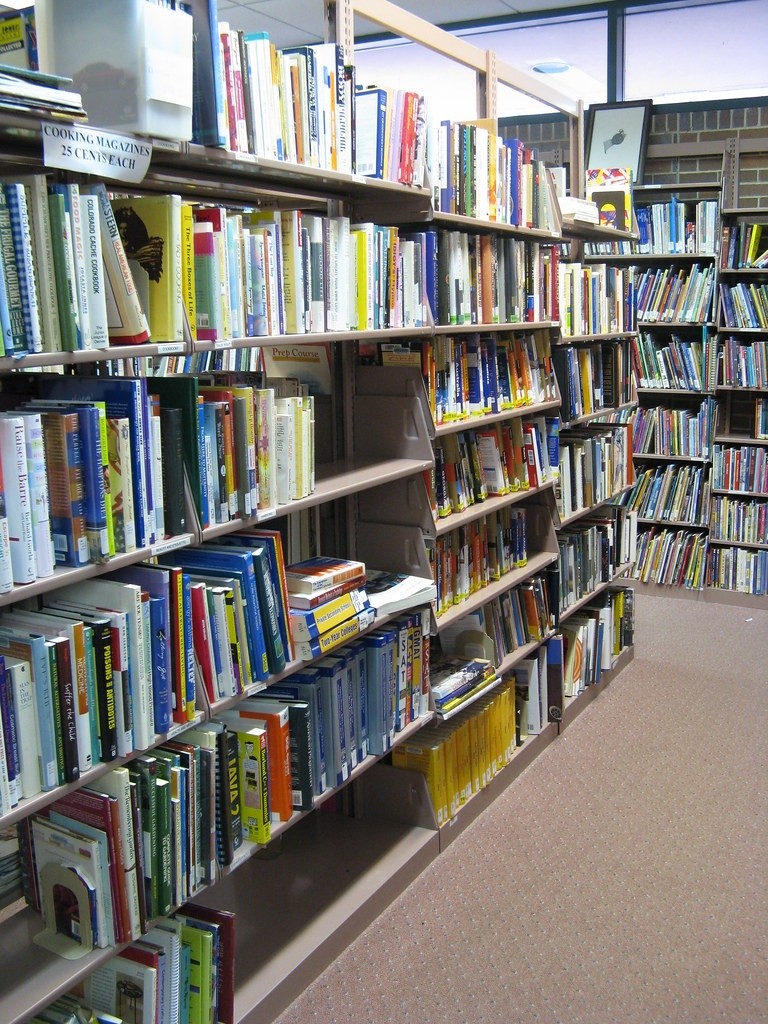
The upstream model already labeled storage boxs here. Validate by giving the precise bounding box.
[33,0,193,141]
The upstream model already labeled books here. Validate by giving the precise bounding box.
[0,1,767,1024]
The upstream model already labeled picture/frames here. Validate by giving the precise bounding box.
[584,98,654,186]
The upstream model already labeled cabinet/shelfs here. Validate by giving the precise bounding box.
[0,0,768,1024]
